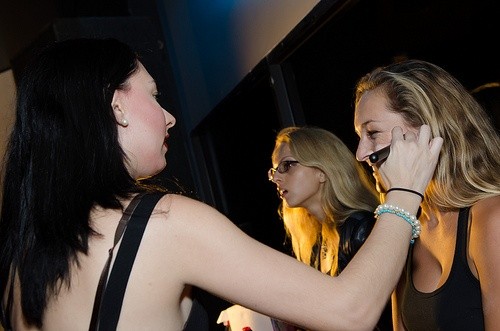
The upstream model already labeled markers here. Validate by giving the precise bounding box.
[368,144,390,164]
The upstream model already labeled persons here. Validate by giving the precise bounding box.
[352,60,499,331]
[0,27,444,331]
[1,52,18,192]
[268,126,397,331]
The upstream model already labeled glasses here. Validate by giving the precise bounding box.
[268,160,299,181]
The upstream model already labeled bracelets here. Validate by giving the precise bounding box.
[373,204,422,244]
[384,187,425,204]
